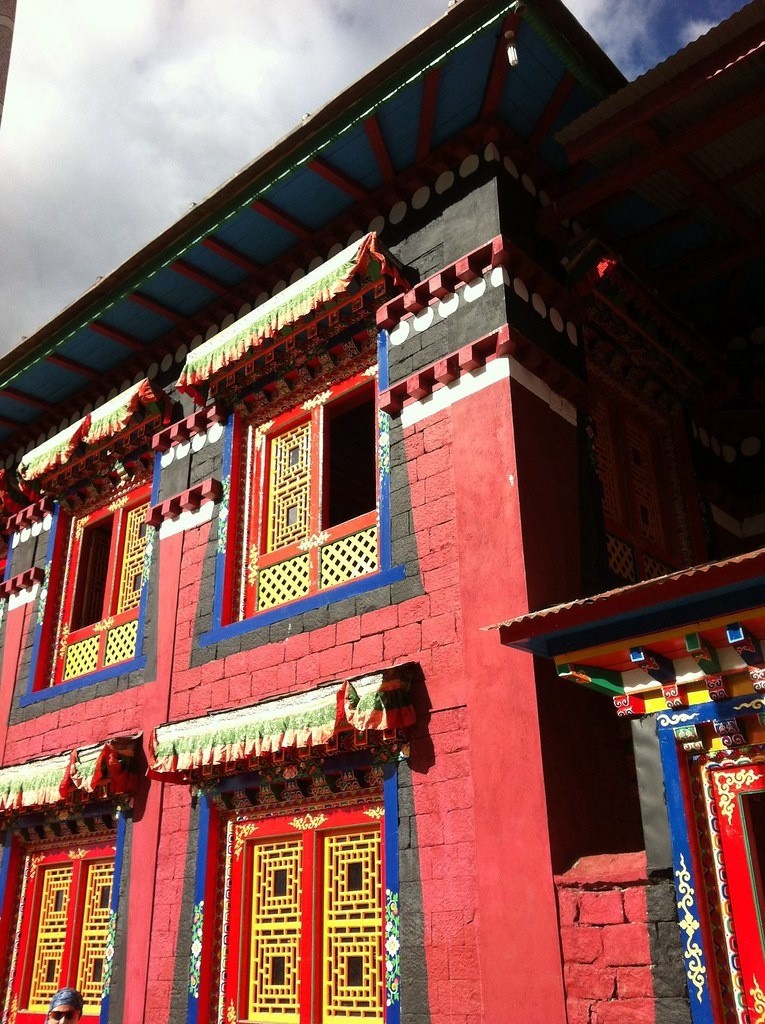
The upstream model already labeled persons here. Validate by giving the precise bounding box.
[45,988,83,1024]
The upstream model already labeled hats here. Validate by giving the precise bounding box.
[49,987,84,1016]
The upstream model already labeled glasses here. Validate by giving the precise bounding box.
[49,1008,79,1020]
